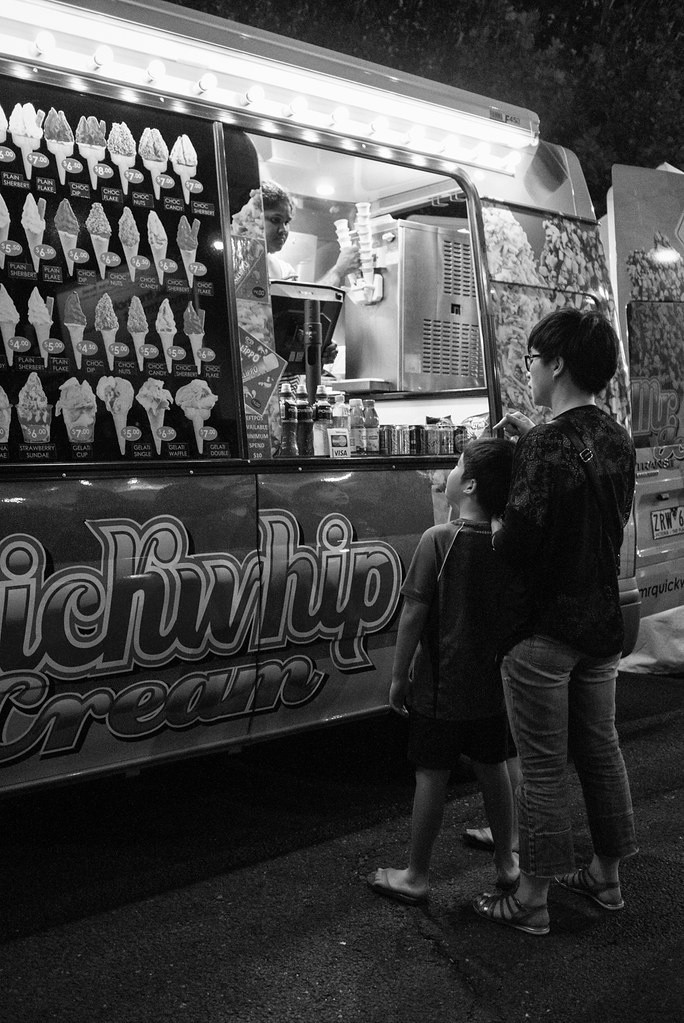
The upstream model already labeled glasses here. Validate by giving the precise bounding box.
[524,354,545,372]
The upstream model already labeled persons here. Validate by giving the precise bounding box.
[260,177,363,393]
[365,437,519,904]
[471,307,641,936]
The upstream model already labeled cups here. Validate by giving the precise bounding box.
[60,405,99,444]
[15,404,52,444]
[0,403,12,443]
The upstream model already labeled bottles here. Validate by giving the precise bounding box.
[272,383,380,458]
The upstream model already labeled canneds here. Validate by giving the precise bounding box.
[379,423,464,456]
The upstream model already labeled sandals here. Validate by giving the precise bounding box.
[472,895,551,935]
[555,868,625,910]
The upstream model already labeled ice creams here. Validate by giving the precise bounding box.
[0,102,218,455]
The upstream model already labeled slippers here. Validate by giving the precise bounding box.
[366,869,427,904]
[465,826,520,853]
